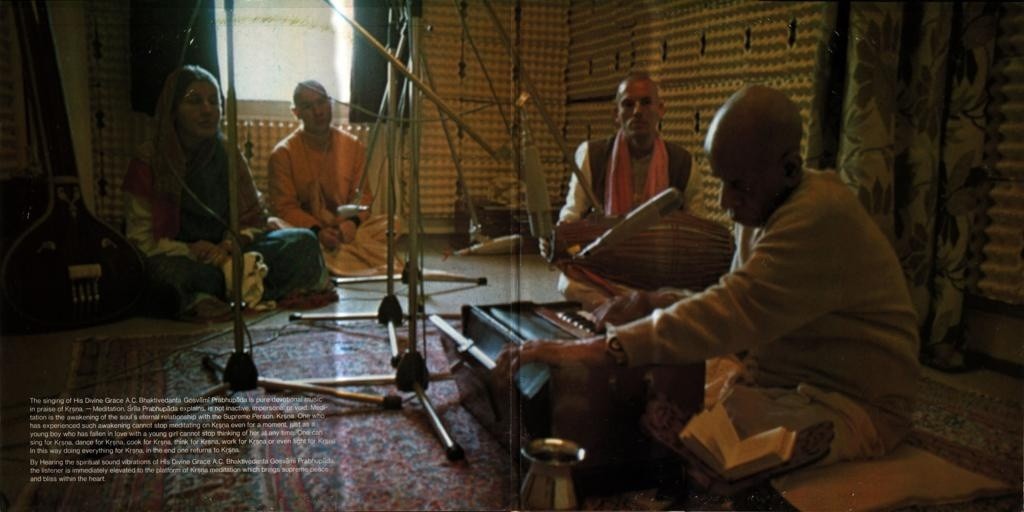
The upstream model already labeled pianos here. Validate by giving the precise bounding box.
[429,299,705,494]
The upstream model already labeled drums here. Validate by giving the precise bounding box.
[544,216,734,291]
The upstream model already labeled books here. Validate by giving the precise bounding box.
[675,399,800,483]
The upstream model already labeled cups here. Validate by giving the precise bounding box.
[517,435,588,512]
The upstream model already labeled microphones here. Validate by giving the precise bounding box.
[453,235,523,255]
[336,203,369,215]
[519,129,553,238]
[571,186,683,262]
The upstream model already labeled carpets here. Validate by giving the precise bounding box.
[6,317,1023,512]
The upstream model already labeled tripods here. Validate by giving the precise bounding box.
[85,0,400,463]
[265,0,465,463]
[327,0,487,312]
[287,0,465,371]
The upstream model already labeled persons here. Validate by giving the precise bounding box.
[120,65,332,325]
[555,68,694,308]
[496,83,920,492]
[264,79,403,278]
